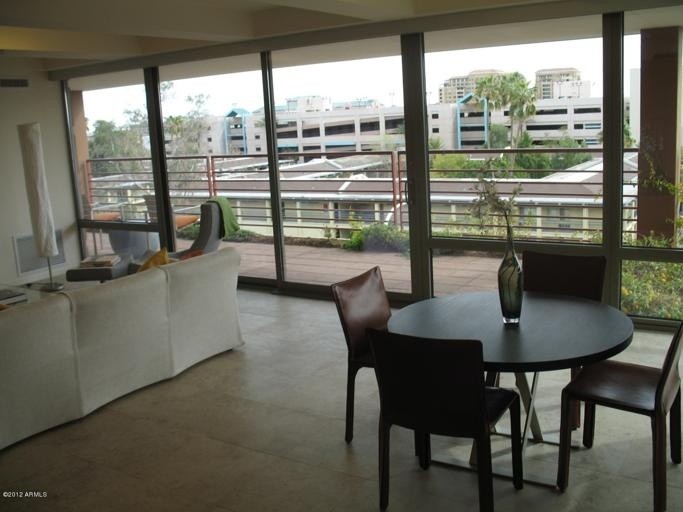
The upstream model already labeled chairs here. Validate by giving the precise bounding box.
[127,196,227,274]
[328,248,681,511]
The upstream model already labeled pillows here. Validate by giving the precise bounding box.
[136,247,169,271]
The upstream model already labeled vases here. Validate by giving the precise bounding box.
[496,227,523,325]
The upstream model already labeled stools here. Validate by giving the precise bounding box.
[65,253,132,285]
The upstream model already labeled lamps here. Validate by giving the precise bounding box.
[17,122,65,293]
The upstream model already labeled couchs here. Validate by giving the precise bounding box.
[1,247,243,446]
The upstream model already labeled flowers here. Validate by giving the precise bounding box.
[467,167,528,230]
